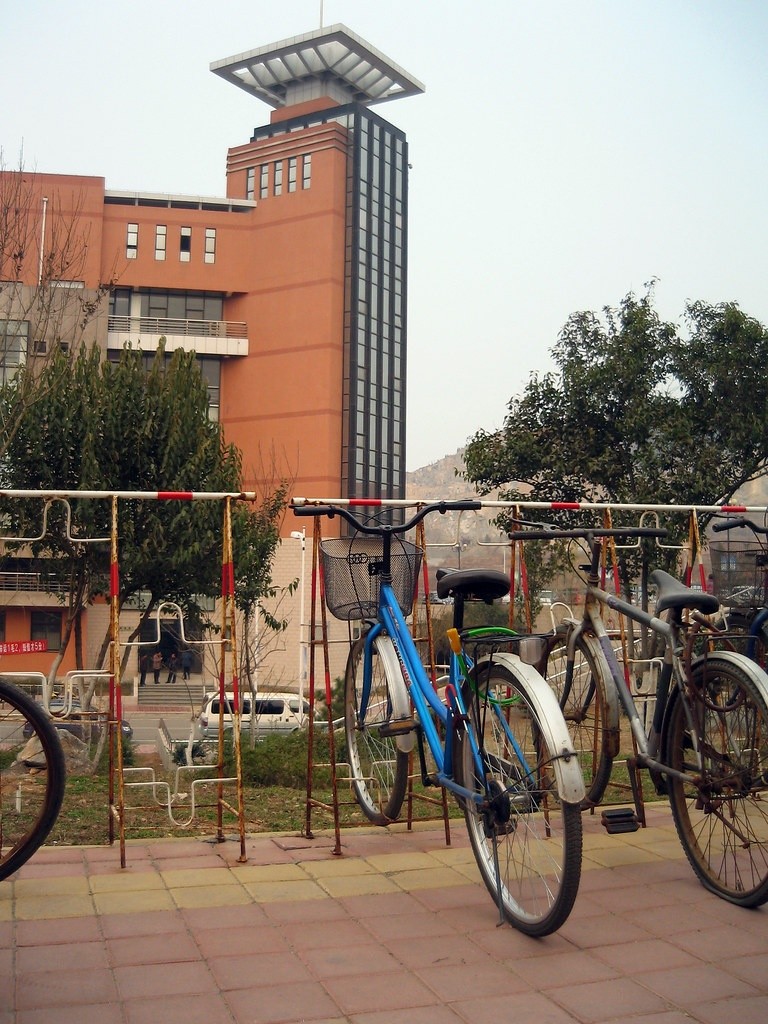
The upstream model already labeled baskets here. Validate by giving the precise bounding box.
[319,537,424,621]
[708,541,768,607]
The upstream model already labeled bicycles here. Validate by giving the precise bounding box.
[287,495,593,937]
[704,511,768,698]
[508,526,767,907]
[0,674,65,882]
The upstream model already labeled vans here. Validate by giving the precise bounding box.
[202,692,324,742]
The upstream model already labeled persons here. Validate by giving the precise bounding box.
[165,654,178,683]
[140,652,148,687]
[182,651,192,680]
[152,651,163,684]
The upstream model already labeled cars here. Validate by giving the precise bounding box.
[423,584,765,606]
[24,698,136,741]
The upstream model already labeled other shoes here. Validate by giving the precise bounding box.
[172,681,175,683]
[140,684,145,686]
[166,681,170,683]
